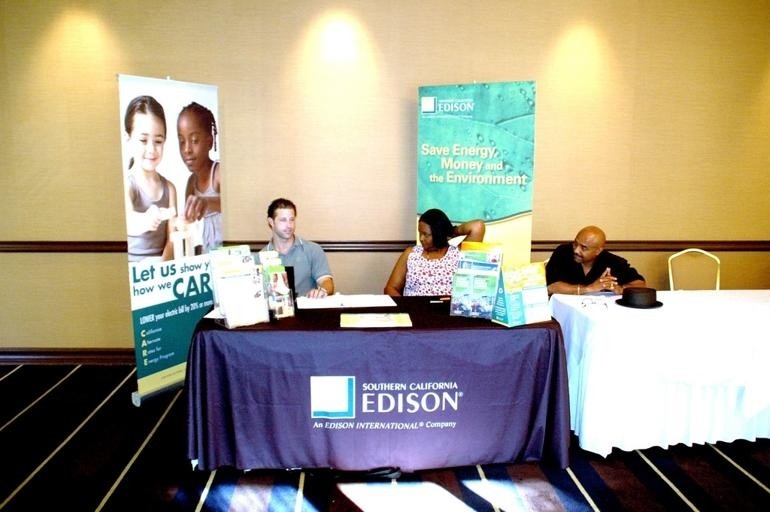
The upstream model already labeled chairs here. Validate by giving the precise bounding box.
[667,248,722,289]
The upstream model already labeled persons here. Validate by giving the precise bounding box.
[122,95,177,265]
[384,209,485,297]
[177,101,223,254]
[546,226,647,296]
[258,198,334,299]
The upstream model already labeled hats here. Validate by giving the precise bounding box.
[616,287,663,308]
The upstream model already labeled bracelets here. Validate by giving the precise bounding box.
[577,284,580,295]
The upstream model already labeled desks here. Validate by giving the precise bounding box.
[183,293,570,474]
[552,286,770,456]
